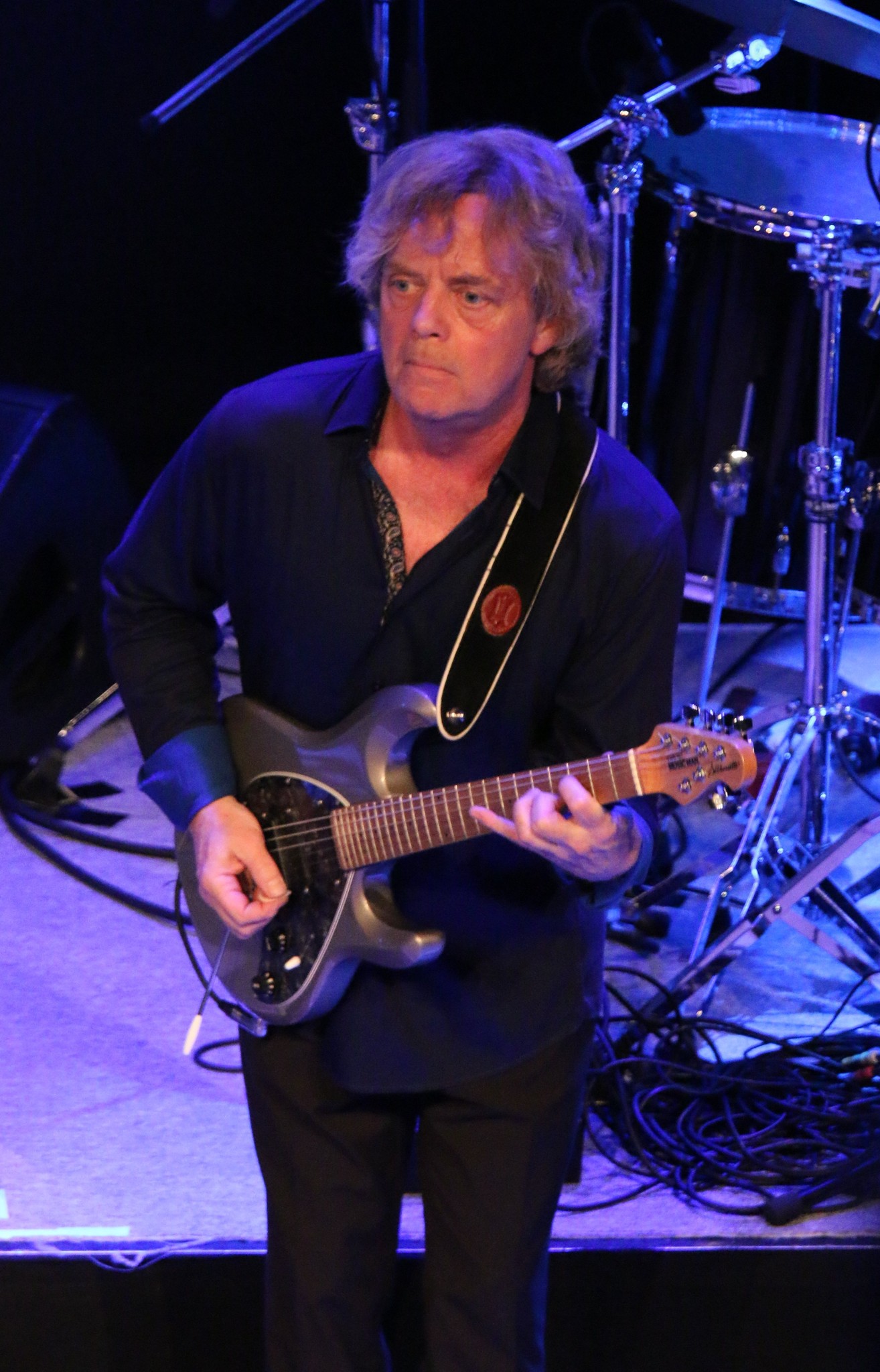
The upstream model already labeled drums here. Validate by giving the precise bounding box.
[584,110,880,625]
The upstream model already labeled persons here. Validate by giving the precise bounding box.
[100,130,685,1372]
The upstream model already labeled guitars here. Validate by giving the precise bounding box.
[173,687,757,1026]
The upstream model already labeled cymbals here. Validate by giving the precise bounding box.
[682,0,880,80]
